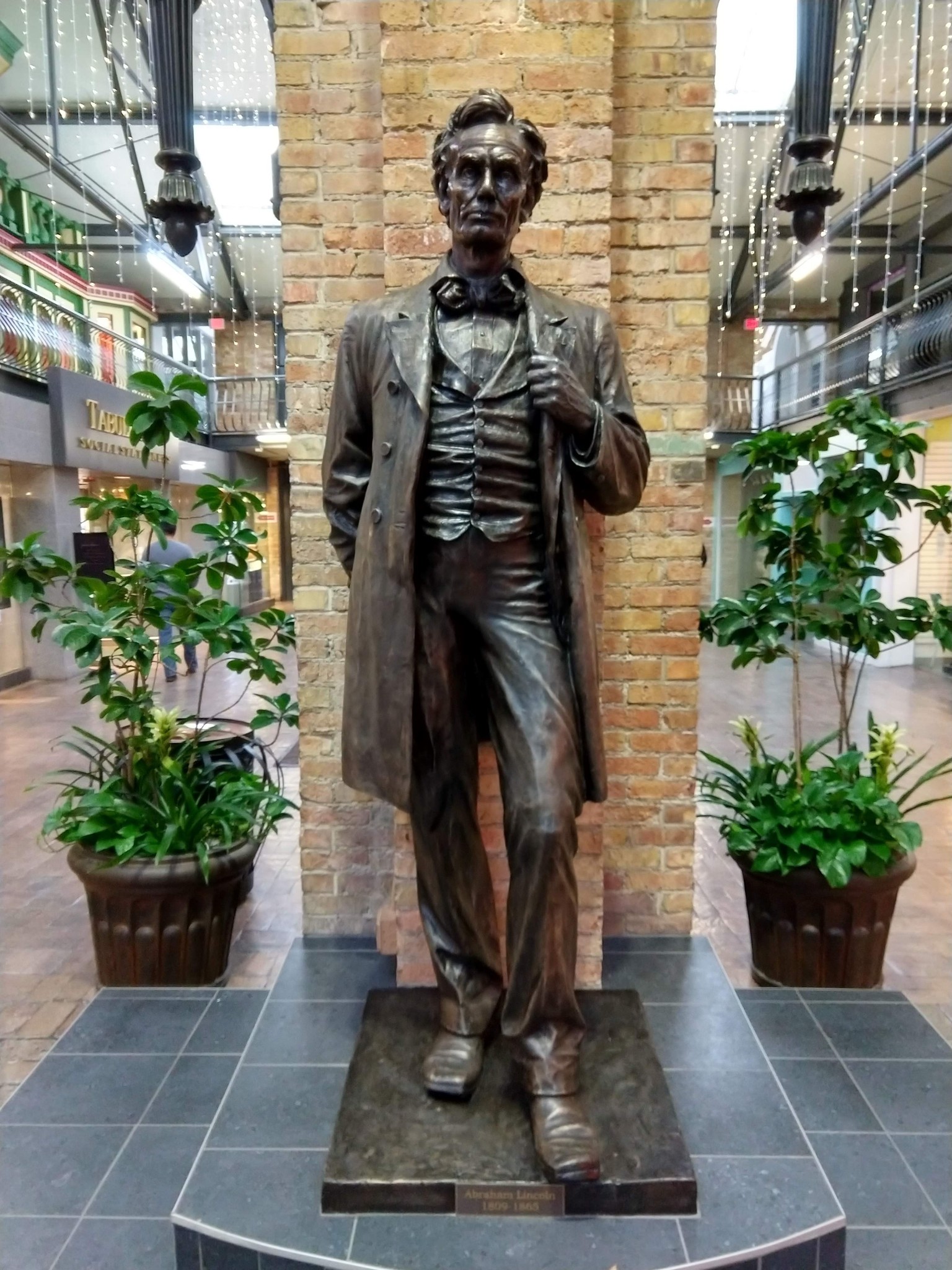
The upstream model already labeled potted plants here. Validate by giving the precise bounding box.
[0,370,298,989]
[693,383,952,989]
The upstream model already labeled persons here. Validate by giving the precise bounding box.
[320,88,649,1182]
[140,520,200,683]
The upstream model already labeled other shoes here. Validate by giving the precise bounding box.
[186,669,196,675]
[166,675,177,682]
